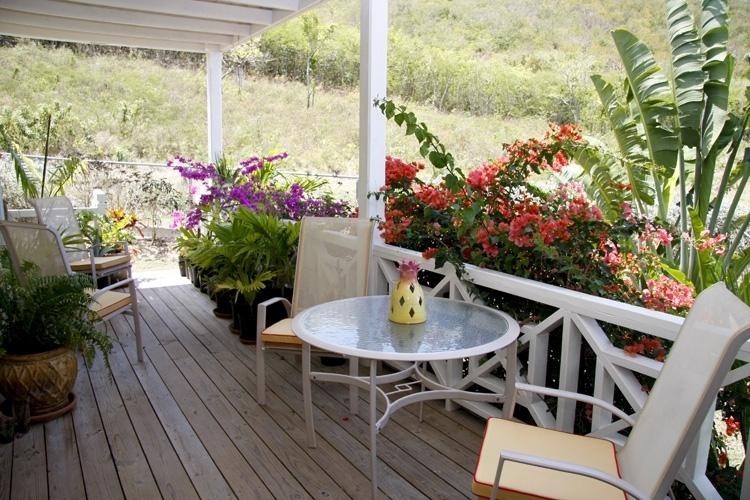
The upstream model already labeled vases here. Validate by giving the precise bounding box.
[320,357,346,368]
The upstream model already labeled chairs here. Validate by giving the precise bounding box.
[1,220,143,364]
[255,217,375,414]
[26,196,132,288]
[471,281,749,500]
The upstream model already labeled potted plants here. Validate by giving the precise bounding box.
[0,248,113,425]
[174,203,302,345]
[82,208,146,290]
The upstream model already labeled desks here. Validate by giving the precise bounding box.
[290,295,520,500]
